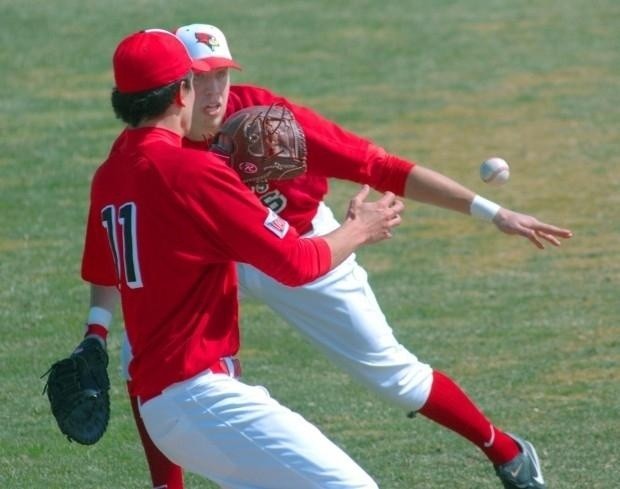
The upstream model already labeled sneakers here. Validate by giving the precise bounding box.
[491,429,545,489]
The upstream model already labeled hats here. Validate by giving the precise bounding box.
[113,28,212,94]
[171,24,244,75]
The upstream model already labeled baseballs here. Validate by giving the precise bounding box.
[479,158,510,185]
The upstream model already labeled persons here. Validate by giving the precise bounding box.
[40,23,573,489]
[93,26,404,489]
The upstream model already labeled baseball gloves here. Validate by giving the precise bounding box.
[209,106,307,182]
[41,339,110,445]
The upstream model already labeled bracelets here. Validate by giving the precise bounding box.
[470,193,501,223]
